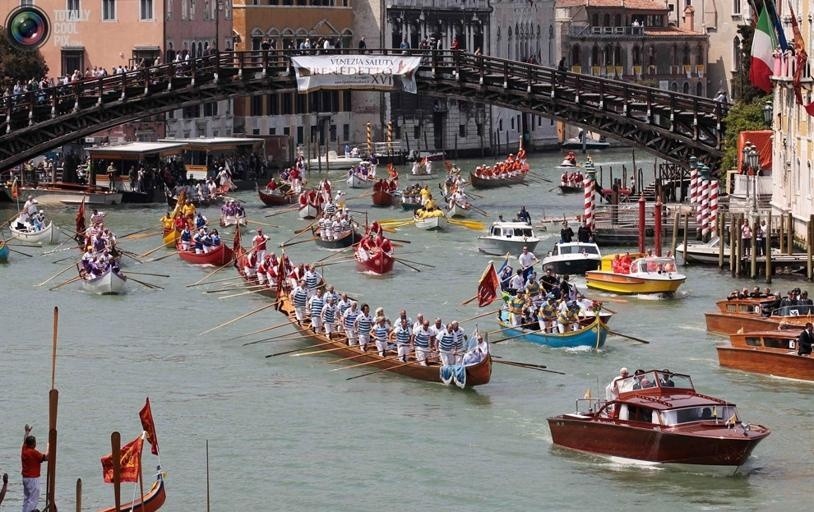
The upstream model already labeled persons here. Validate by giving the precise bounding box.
[605,366,713,423]
[236,226,321,294]
[0,472,9,505]
[2,41,217,110]
[528,53,567,87]
[741,218,768,257]
[20,423,48,512]
[475,146,635,202]
[284,262,487,367]
[266,152,470,262]
[0,145,266,279]
[496,201,694,334]
[259,32,484,73]
[711,88,732,117]
[724,285,814,355]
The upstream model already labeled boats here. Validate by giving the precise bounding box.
[298,152,473,281]
[475,218,540,258]
[1,136,305,299]
[278,286,493,391]
[534,237,604,278]
[546,368,772,467]
[677,236,783,272]
[92,466,169,512]
[494,275,615,352]
[704,289,814,387]
[469,138,636,202]
[583,251,688,297]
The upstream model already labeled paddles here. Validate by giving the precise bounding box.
[291,344,360,356]
[115,220,182,290]
[313,243,357,268]
[227,319,300,340]
[386,238,412,244]
[346,209,367,214]
[198,283,327,338]
[389,256,421,273]
[327,350,393,364]
[325,286,358,303]
[373,179,487,230]
[241,328,323,349]
[264,337,344,359]
[190,238,268,286]
[494,168,553,188]
[388,255,435,268]
[346,356,442,380]
[246,219,285,229]
[461,259,649,375]
[328,353,416,372]
[264,203,329,247]
[186,273,280,298]
[0,208,85,291]
[349,218,371,230]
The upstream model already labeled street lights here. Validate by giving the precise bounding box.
[740,140,761,215]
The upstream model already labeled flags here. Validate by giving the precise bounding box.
[138,396,158,457]
[748,3,808,106]
[100,432,143,484]
[476,262,499,308]
